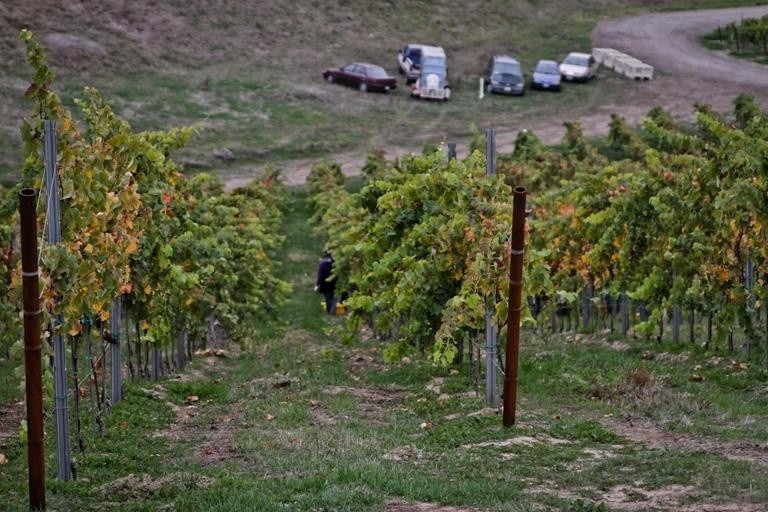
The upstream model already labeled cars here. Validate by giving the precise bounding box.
[323,60,397,93]
[528,58,561,90]
[560,50,599,82]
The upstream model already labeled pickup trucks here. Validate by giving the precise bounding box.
[396,43,433,84]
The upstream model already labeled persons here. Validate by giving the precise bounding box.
[315,251,339,315]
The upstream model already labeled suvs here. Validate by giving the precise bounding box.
[410,45,451,99]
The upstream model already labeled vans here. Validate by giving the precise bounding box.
[484,53,524,96]
